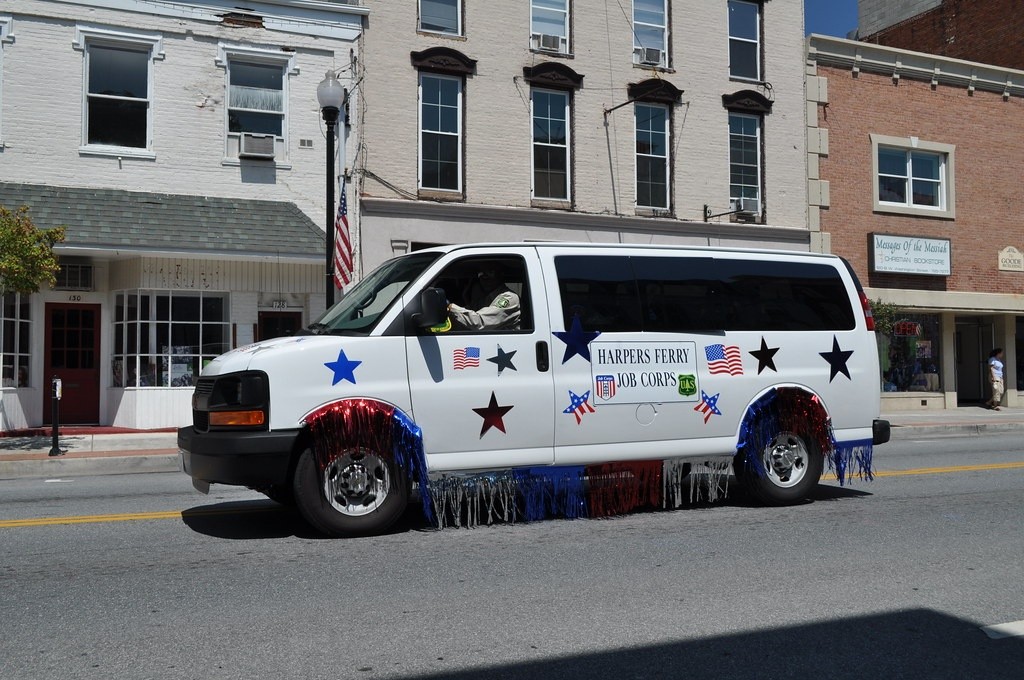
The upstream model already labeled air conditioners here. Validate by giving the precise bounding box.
[237,131,276,160]
[735,196,759,215]
[538,33,561,52]
[639,47,662,65]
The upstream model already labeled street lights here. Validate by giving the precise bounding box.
[312,70,347,310]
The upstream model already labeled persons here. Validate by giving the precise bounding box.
[985,347,1005,411]
[443,264,524,333]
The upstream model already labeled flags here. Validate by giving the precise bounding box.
[329,178,356,289]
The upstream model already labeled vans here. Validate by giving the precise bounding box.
[174,227,890,536]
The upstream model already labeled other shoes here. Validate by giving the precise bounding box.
[991,405,1000,410]
[985,401,992,406]
